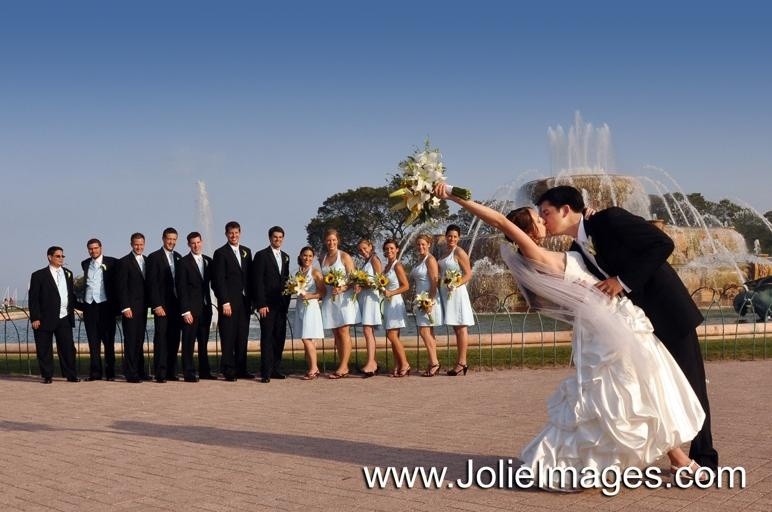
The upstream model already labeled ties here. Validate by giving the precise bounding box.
[56,270,61,286]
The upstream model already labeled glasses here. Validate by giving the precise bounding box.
[53,253,66,258]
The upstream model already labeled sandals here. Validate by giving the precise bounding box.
[329,369,350,378]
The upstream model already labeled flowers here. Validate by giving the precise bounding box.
[282,265,463,325]
[382,134,472,229]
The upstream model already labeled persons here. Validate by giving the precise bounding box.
[539,185,719,479]
[433,182,715,494]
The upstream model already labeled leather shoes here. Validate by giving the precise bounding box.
[45,377,52,383]
[106,377,114,381]
[199,373,217,380]
[184,376,198,382]
[226,371,286,383]
[85,376,102,381]
[127,375,178,383]
[68,375,80,382]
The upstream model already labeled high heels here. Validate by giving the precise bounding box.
[304,368,321,380]
[447,362,468,376]
[670,460,717,486]
[422,361,441,376]
[389,367,410,376]
